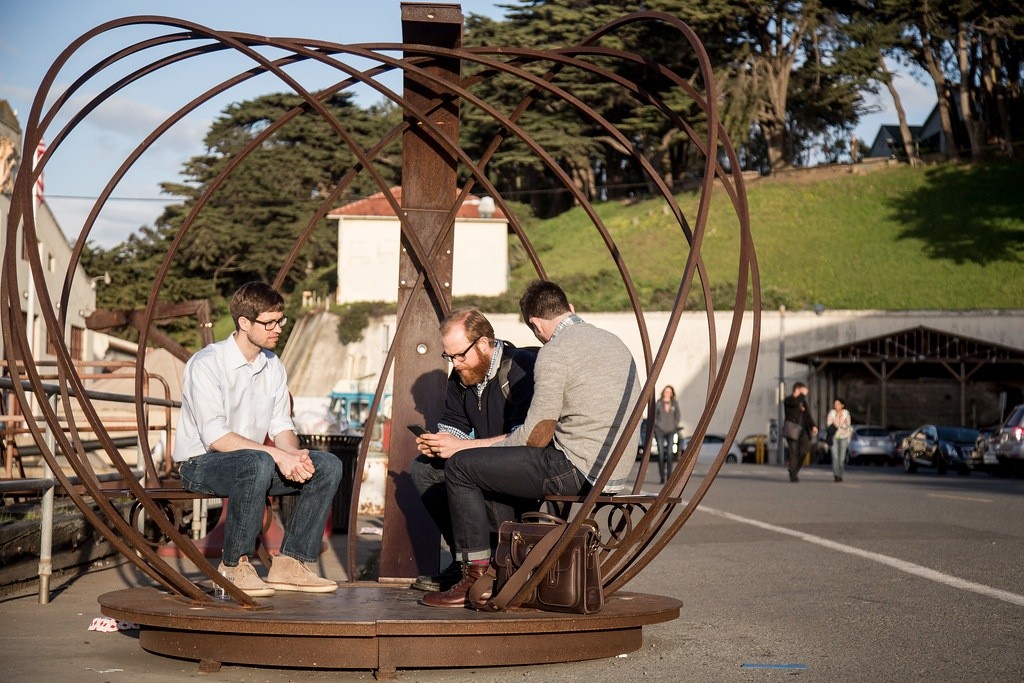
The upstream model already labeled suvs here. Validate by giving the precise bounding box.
[993,405,1024,469]
[635,417,684,462]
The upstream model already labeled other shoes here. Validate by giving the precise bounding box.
[791,474,800,482]
[834,474,841,481]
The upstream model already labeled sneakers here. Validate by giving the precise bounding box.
[267,555,339,593]
[213,556,275,597]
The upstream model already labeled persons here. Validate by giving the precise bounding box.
[425,281,644,607]
[827,398,852,484]
[408,307,536,593]
[783,381,820,483]
[654,385,682,484]
[172,283,345,594]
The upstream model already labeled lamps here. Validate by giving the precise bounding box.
[90,271,111,290]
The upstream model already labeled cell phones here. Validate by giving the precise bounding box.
[406,424,430,437]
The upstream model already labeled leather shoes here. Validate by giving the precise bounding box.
[421,565,490,607]
[412,561,465,591]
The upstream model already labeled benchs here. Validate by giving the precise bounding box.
[82,485,301,572]
[535,494,683,550]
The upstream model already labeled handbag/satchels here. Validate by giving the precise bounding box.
[781,421,802,439]
[494,512,604,614]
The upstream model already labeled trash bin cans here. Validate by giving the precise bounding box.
[285,434,363,536]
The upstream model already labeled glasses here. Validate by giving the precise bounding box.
[238,315,288,331]
[441,335,481,361]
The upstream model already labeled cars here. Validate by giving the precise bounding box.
[731,433,788,465]
[679,434,743,465]
[899,424,983,477]
[847,425,896,467]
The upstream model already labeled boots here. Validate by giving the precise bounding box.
[659,464,665,483]
